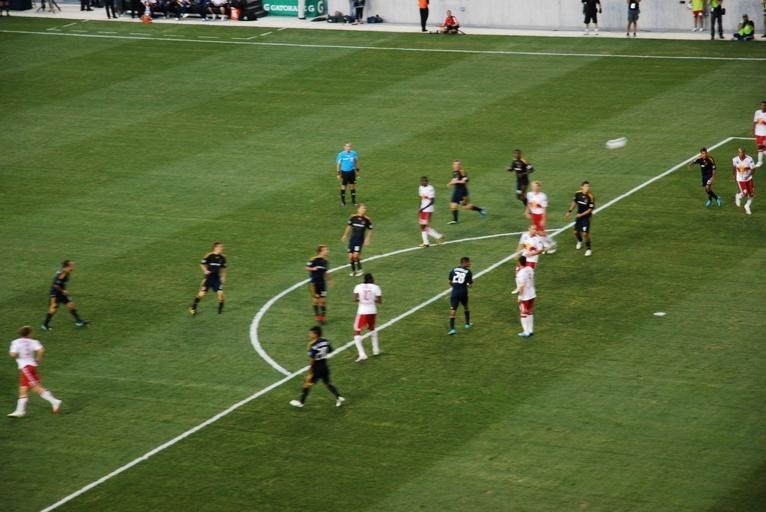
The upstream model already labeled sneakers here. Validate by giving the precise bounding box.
[576,239,583,249]
[417,243,429,248]
[188,306,198,316]
[7,411,26,418]
[355,355,368,362]
[448,220,457,224]
[465,323,473,329]
[706,192,754,215]
[585,249,593,256]
[372,349,380,355]
[447,329,456,335]
[289,400,304,407]
[52,400,62,412]
[336,396,345,407]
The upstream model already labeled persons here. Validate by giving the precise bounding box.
[352,274,382,361]
[2,1,766,43]
[40,258,91,332]
[448,257,474,335]
[751,100,766,168]
[417,176,445,247]
[566,181,595,256]
[6,324,62,419]
[686,146,721,208]
[448,161,487,223]
[508,149,556,338]
[337,143,360,206]
[731,145,756,215]
[343,204,373,275]
[289,325,344,407]
[187,240,228,318]
[305,245,331,326]
